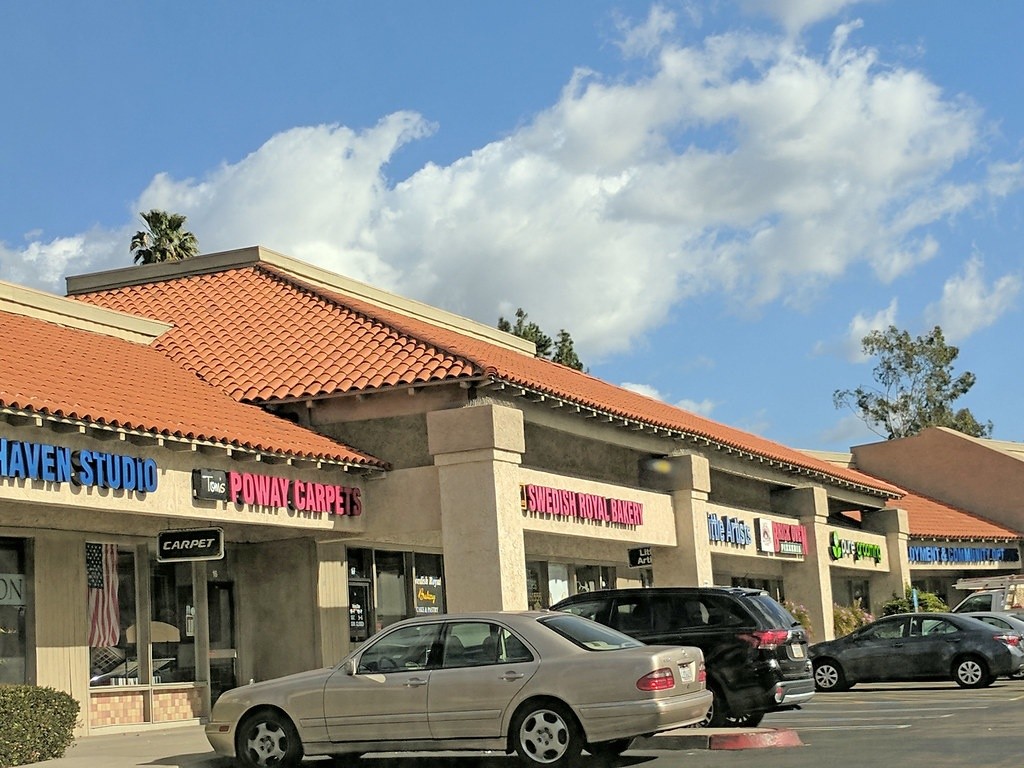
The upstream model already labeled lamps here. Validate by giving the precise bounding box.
[645,456,673,476]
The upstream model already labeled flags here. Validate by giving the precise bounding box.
[85,543,119,646]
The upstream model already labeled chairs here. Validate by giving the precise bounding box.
[480,634,504,662]
[444,636,467,667]
[629,606,649,631]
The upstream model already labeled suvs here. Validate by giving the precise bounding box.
[466,586,816,729]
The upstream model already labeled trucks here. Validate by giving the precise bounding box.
[950,589,1024,622]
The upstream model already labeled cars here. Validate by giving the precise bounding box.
[808,612,1024,692]
[206,610,714,768]
[923,613,1024,680]
